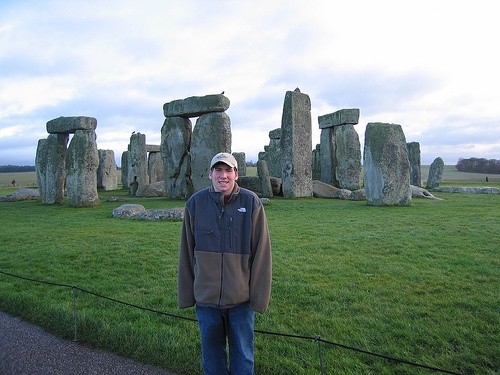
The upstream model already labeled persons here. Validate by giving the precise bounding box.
[485,176,489,182]
[11,178,16,187]
[177,152,272,375]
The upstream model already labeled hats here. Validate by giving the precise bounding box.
[210,152,238,171]
